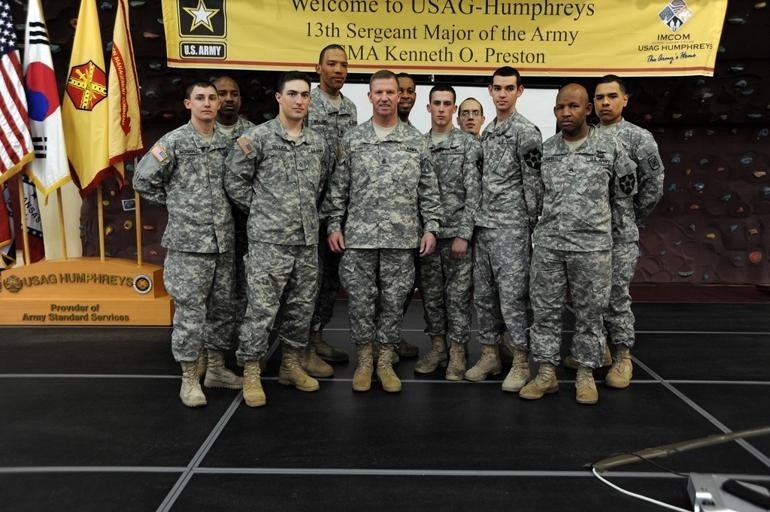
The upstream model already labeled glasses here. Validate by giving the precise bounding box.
[458,110,483,117]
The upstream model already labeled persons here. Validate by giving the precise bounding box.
[328,70,446,393]
[456,97,485,141]
[519,85,637,404]
[591,75,664,389]
[132,79,243,407]
[301,45,356,363]
[213,77,257,367]
[224,71,330,407]
[465,66,544,393]
[394,73,416,130]
[414,83,483,380]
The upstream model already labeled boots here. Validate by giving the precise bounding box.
[376,343,402,392]
[563,344,613,368]
[179,361,208,408]
[574,367,599,404]
[203,351,243,389]
[445,340,467,381]
[242,361,266,407]
[605,346,633,389]
[351,342,374,391]
[502,354,532,392]
[313,331,350,362]
[518,362,560,400]
[464,344,502,383]
[413,335,447,374]
[305,351,334,377]
[278,350,320,392]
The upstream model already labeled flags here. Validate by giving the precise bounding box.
[0,173,45,266]
[24,0,72,262]
[62,0,108,261]
[0,0,35,266]
[108,0,148,266]
